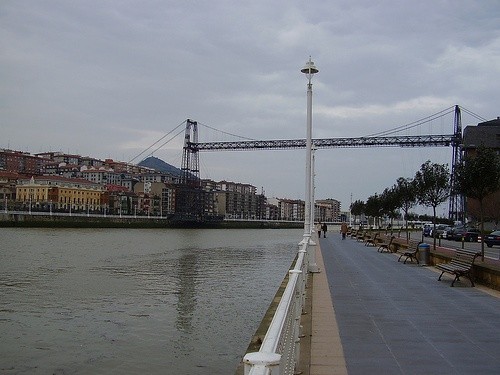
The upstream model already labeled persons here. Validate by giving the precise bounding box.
[341,222,349,241]
[317,222,327,238]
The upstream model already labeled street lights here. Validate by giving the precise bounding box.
[310,141,316,231]
[301,56,319,236]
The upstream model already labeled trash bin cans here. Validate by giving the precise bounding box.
[419,243,430,267]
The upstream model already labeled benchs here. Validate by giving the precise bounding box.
[377,235,395,252]
[347,228,354,236]
[357,231,367,242]
[364,232,378,247]
[398,241,422,264]
[435,248,478,287]
[351,230,359,238]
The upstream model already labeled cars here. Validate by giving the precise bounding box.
[423,224,481,242]
[484,231,500,247]
